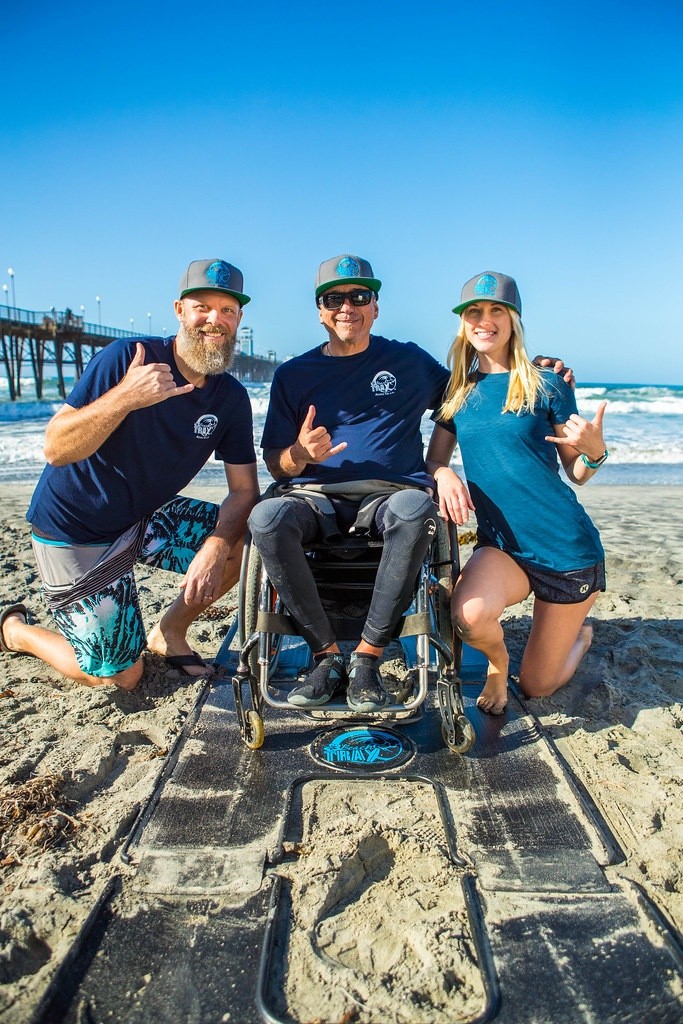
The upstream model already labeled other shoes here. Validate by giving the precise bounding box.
[346,651,388,713]
[287,651,346,706]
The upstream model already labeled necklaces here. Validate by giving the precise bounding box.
[325,344,334,357]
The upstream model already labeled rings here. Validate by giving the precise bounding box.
[205,596,213,600]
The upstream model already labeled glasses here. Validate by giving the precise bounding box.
[319,289,376,310]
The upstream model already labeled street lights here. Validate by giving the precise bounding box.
[163,325,167,339]
[2,284,11,322]
[8,266,18,321]
[96,295,104,335]
[80,305,87,324]
[148,313,152,333]
[130,317,135,335]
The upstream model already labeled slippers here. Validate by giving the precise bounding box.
[166,650,206,676]
[0,603,28,653]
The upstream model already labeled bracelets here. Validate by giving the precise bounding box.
[581,450,608,469]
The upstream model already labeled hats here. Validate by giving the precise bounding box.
[451,270,522,316]
[180,260,251,306]
[314,254,382,310]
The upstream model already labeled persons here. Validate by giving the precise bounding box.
[425,270,610,715]
[0,259,260,692]
[246,253,576,712]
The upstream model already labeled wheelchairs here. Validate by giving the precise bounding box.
[229,477,477,760]
